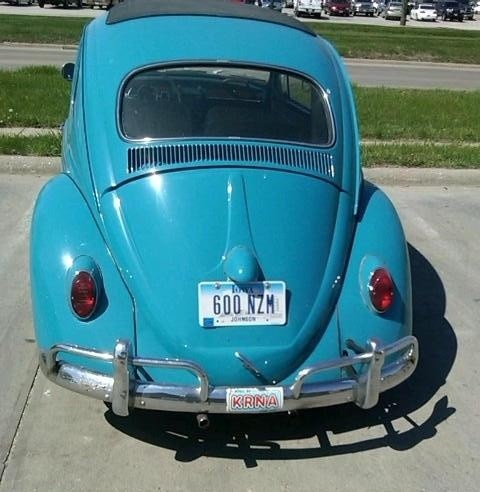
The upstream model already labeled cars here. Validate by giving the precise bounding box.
[254,0,480,26]
[28,0,420,428]
[0,0,121,10]
